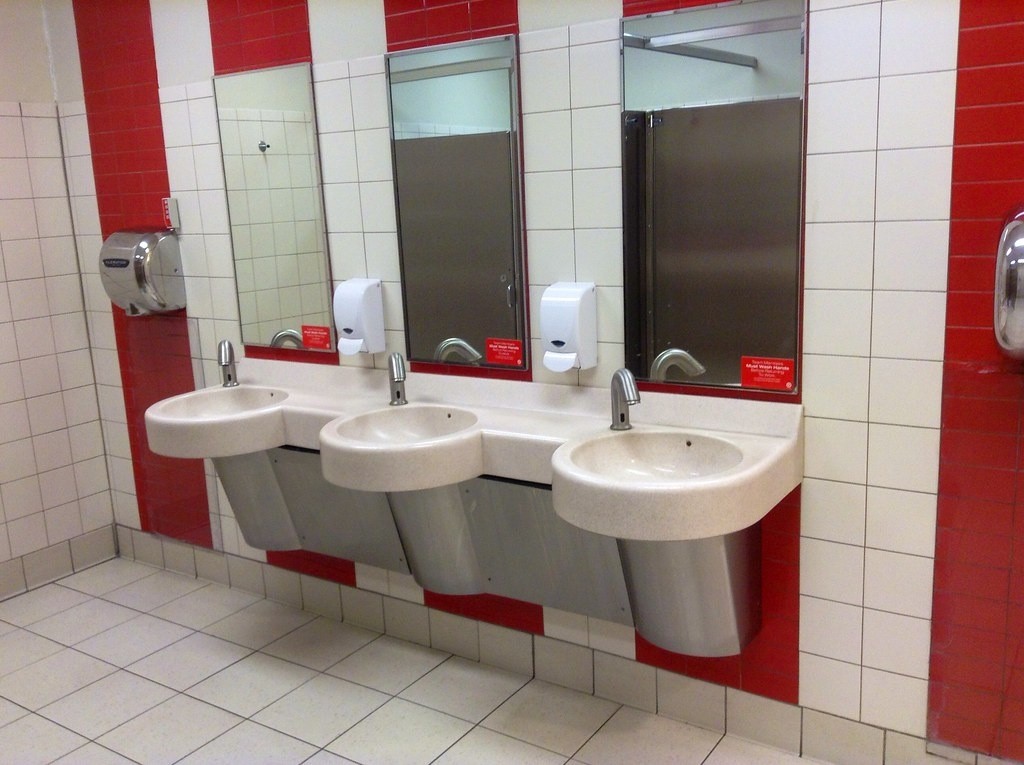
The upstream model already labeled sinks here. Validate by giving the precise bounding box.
[552,428,756,542]
[143,384,289,459]
[318,404,482,492]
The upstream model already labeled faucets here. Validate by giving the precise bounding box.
[217,339,240,387]
[608,367,642,431]
[433,337,486,367]
[649,348,707,384]
[269,330,308,350]
[387,353,409,408]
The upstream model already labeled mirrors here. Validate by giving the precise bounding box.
[384,34,528,372]
[212,63,338,352]
[618,0,809,395]
